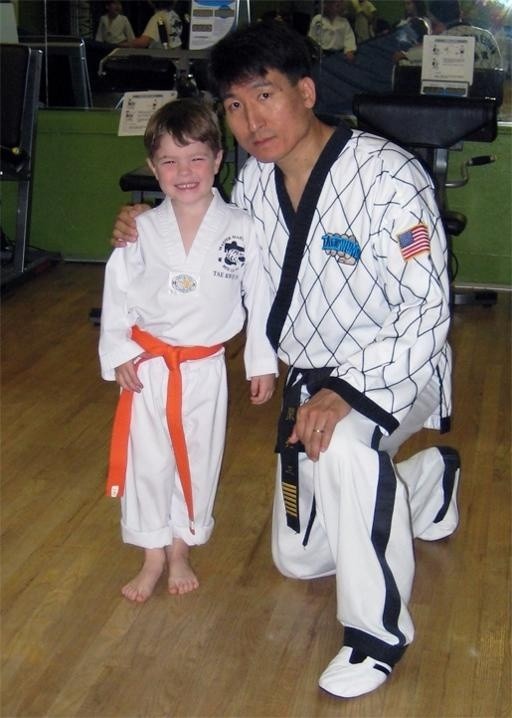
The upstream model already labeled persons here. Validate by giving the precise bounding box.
[306,0,505,73]
[116,0,185,52]
[94,0,135,44]
[109,19,463,697]
[94,97,280,604]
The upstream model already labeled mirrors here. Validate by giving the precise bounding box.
[14,3,510,129]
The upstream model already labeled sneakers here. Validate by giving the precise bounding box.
[416,468,459,540]
[318,645,393,698]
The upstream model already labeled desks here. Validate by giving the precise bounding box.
[98,48,218,110]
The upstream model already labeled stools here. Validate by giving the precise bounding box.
[349,94,498,310]
[121,165,166,204]
